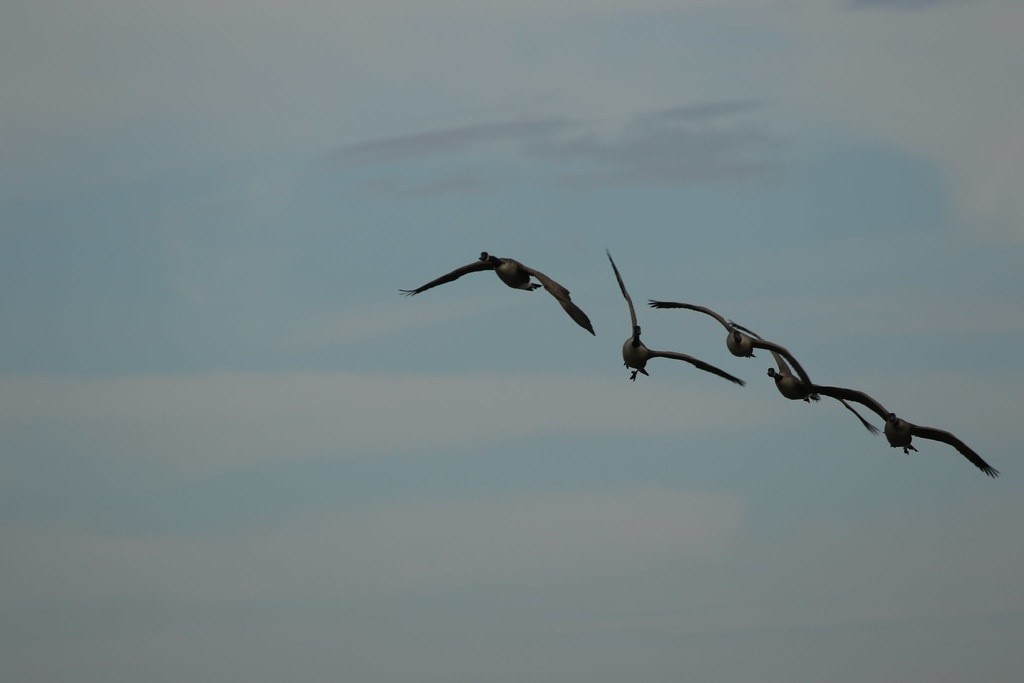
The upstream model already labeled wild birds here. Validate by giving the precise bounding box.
[725,319,881,436]
[837,388,1001,480]
[398,251,597,338]
[647,299,823,402]
[604,247,747,387]
